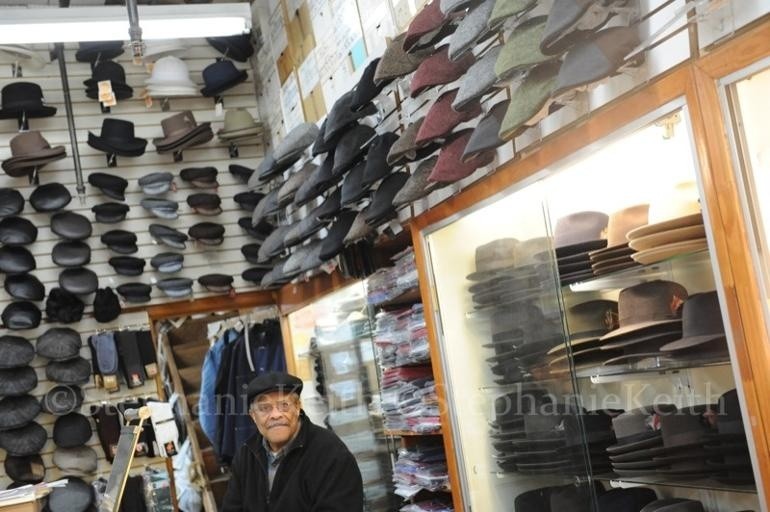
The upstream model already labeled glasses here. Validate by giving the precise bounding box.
[248,402,297,414]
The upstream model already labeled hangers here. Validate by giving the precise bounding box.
[203,309,277,342]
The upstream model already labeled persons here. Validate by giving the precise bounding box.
[217,368,365,512]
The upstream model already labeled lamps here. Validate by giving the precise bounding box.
[0,1,254,45]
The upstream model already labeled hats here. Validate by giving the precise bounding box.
[247,370,304,401]
[493,11,558,79]
[486,0,541,31]
[498,58,573,142]
[539,1,604,58]
[551,26,644,96]
[1,327,97,512]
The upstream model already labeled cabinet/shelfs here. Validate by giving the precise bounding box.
[280,11,770,512]
